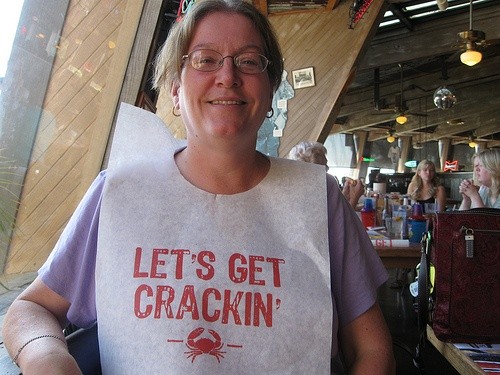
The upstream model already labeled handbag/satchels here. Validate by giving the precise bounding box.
[392,207,500,375]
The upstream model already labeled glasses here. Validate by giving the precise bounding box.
[182,49,270,74]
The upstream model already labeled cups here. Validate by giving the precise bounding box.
[394,212,403,237]
[424,203,439,214]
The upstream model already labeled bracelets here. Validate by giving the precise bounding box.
[12,335,66,368]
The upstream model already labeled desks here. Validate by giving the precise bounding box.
[368,228,422,269]
[425,324,500,375]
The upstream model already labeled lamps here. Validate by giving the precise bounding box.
[386,137,395,142]
[460,50,483,67]
[396,116,408,124]
[469,141,477,148]
[433,85,458,111]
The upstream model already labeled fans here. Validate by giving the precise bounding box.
[373,124,408,137]
[371,64,428,119]
[451,132,496,145]
[412,0,500,64]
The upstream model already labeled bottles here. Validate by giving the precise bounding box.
[416,260,435,294]
[361,187,429,243]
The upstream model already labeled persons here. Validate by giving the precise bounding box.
[287,141,365,212]
[3,0,393,375]
[458,148,500,210]
[407,159,446,211]
